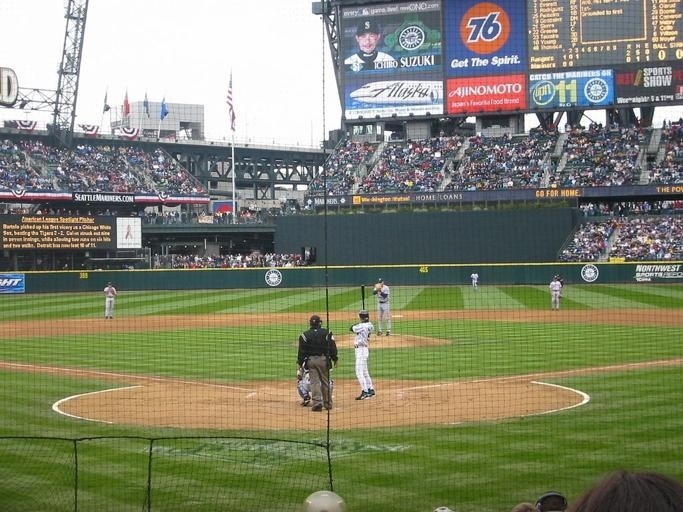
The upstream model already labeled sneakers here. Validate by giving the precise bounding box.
[300,394,311,405]
[354,389,375,399]
[312,402,322,411]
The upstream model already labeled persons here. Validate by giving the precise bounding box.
[557,274,564,288]
[344,18,396,70]
[154,251,302,268]
[16,207,116,216]
[101,281,117,320]
[296,313,337,413]
[350,309,376,402]
[294,362,334,408]
[546,275,562,311]
[311,119,683,197]
[131,211,270,225]
[0,137,204,197]
[372,276,391,336]
[468,270,478,288]
[566,469,682,512]
[561,200,683,262]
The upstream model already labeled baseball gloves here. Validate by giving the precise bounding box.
[375,284,382,292]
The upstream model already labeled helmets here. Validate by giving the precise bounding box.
[309,315,322,325]
[358,309,369,320]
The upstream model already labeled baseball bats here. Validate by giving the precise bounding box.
[361,284,365,310]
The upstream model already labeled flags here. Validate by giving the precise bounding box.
[160,97,168,120]
[226,73,236,131]
[142,93,150,118]
[124,93,130,117]
[102,91,110,114]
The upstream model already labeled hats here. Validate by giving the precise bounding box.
[377,277,384,283]
